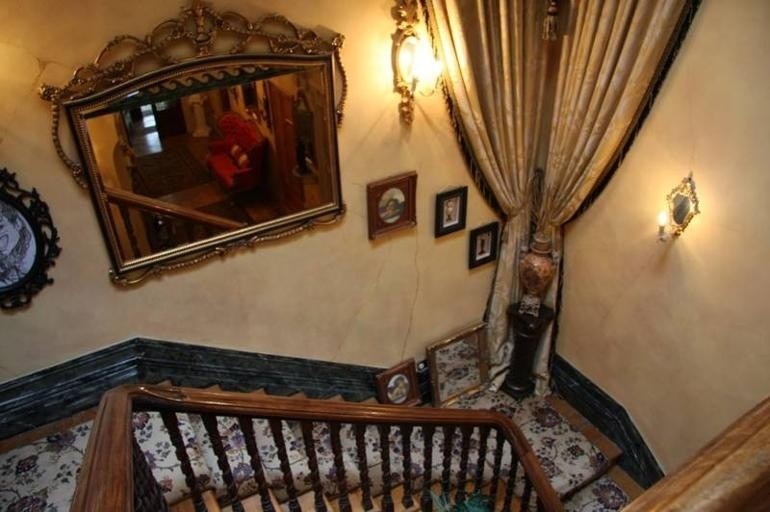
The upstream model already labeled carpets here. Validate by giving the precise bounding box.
[1,389,630,512]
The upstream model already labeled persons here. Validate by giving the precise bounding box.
[385,198,400,218]
[446,199,455,223]
[390,376,407,399]
[478,237,488,257]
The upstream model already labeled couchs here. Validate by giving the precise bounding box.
[187,111,268,198]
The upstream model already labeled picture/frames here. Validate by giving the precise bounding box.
[366,171,499,270]
[374,321,491,409]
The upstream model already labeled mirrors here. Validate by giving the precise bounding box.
[37,2,347,288]
